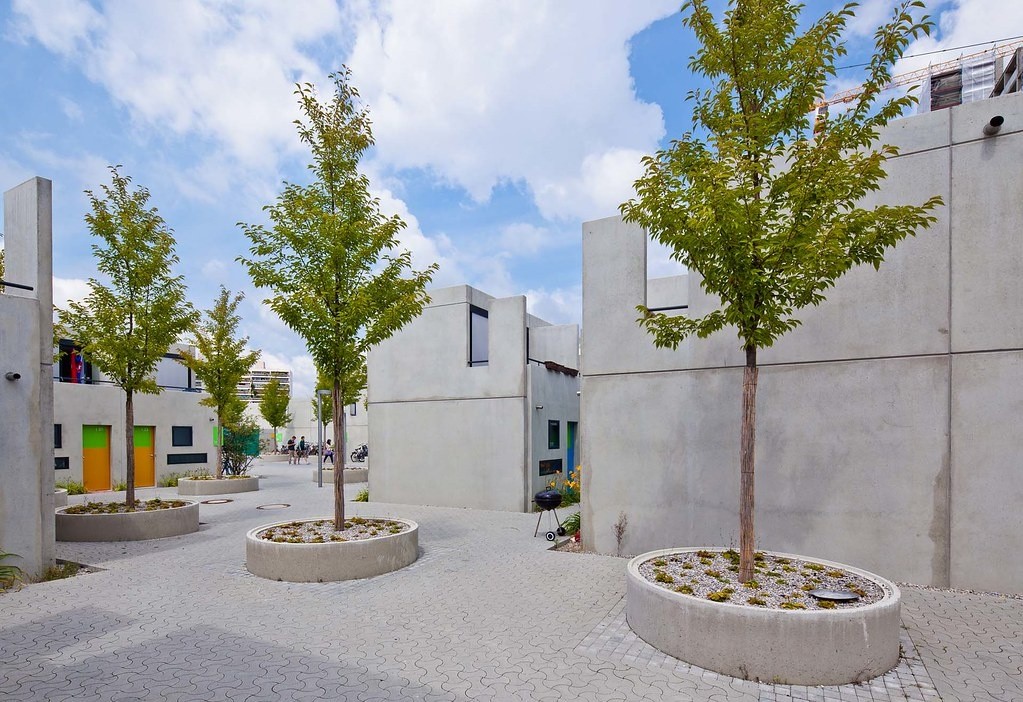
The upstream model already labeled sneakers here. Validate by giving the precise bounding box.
[305,462,311,465]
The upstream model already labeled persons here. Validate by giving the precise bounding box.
[287,436,297,465]
[323,439,335,464]
[297,436,311,465]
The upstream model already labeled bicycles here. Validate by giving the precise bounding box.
[350,442,369,463]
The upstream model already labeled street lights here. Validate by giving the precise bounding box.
[317,389,331,488]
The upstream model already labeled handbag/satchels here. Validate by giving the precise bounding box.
[325,449,332,456]
